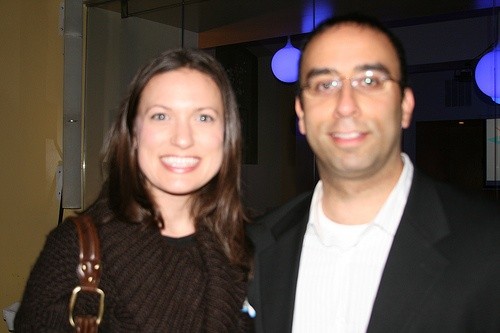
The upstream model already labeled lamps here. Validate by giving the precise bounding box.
[475,39,500,105]
[271,36,303,83]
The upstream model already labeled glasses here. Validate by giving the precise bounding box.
[300,70,400,95]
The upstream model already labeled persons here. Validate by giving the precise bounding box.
[246,16,500,332]
[16,47,257,333]
